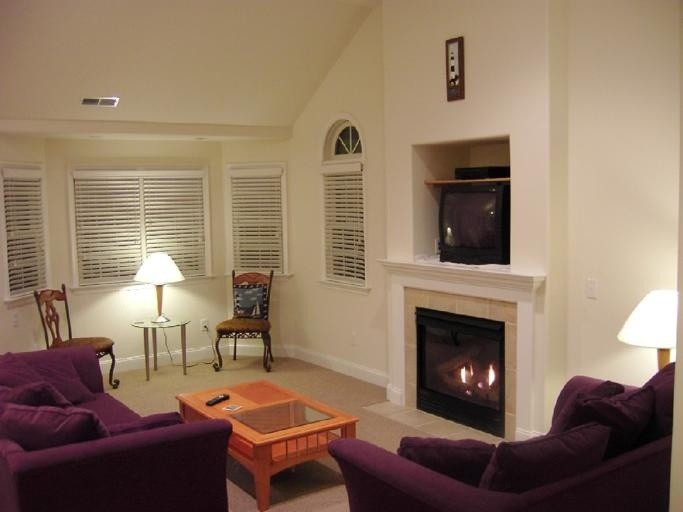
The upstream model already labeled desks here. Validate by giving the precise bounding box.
[129,318,192,383]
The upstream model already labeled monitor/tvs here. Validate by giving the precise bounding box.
[437,182,510,265]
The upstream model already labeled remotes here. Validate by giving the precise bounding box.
[206,393,230,405]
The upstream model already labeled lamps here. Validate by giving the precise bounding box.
[131,250,188,325]
[611,288,678,374]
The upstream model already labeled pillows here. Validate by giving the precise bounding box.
[99,408,183,440]
[232,281,269,322]
[16,345,97,406]
[539,381,628,440]
[394,429,497,490]
[0,349,45,389]
[476,417,613,495]
[0,402,113,452]
[573,382,659,467]
[0,381,77,412]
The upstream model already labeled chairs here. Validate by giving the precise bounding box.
[210,265,278,372]
[30,283,122,389]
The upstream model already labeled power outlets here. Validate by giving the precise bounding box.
[199,318,209,332]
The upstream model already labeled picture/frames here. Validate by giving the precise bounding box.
[443,35,466,103]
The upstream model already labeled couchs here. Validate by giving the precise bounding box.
[320,362,673,512]
[0,344,233,512]
